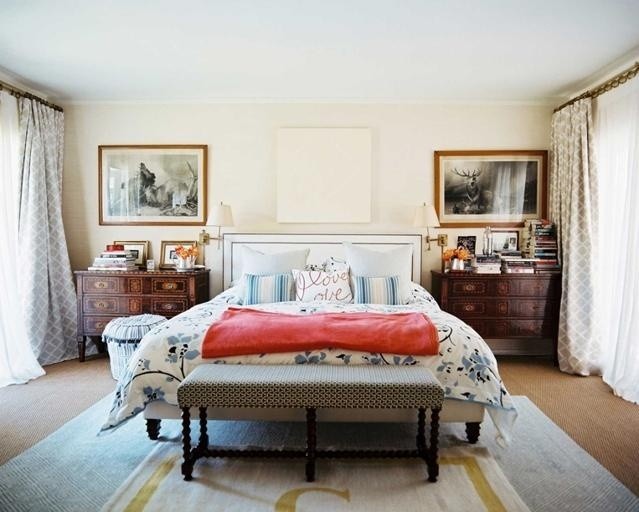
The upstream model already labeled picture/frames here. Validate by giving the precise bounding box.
[434,150,549,229]
[159,240,198,270]
[113,240,150,269]
[98,144,208,227]
[490,231,520,252]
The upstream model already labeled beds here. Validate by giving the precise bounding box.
[96,232,519,447]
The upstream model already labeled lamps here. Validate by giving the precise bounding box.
[198,200,234,250]
[411,203,448,251]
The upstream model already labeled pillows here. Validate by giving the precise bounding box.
[231,247,311,297]
[343,241,415,305]
[291,268,354,303]
[353,273,403,306]
[242,271,296,306]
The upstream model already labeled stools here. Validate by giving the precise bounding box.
[102,314,169,382]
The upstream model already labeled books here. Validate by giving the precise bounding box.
[87,244,140,272]
[472,217,561,275]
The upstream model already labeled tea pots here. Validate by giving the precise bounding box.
[172,253,199,273]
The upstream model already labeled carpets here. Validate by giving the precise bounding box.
[99,435,530,512]
[0,388,639,511]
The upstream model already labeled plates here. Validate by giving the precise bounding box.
[193,264,208,269]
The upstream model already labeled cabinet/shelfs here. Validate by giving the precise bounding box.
[73,269,211,362]
[431,269,562,368]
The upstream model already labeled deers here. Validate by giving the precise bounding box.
[452,167,494,212]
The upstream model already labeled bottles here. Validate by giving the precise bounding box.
[481,226,492,255]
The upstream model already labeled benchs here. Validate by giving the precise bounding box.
[176,363,445,483]
